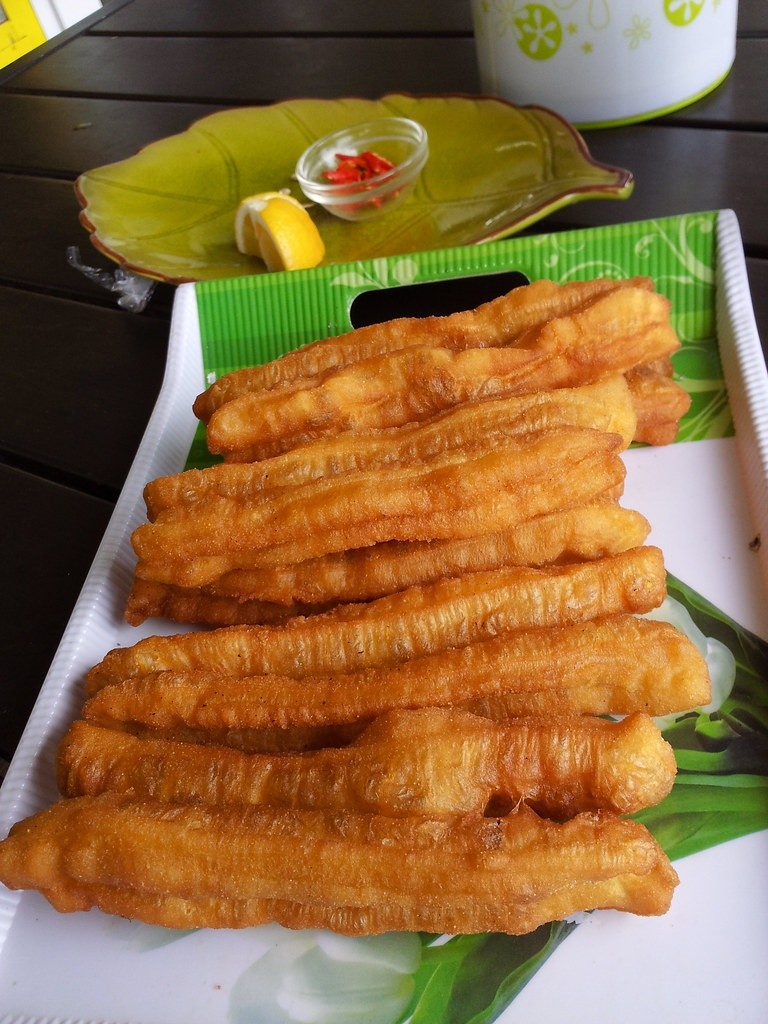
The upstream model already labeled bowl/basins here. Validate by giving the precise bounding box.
[296,117,429,220]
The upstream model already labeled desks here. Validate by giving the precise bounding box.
[0,0,768,1024]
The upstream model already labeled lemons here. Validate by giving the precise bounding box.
[235,191,324,272]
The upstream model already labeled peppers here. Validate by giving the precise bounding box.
[323,152,404,208]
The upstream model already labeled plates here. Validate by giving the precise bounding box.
[74,90,634,282]
